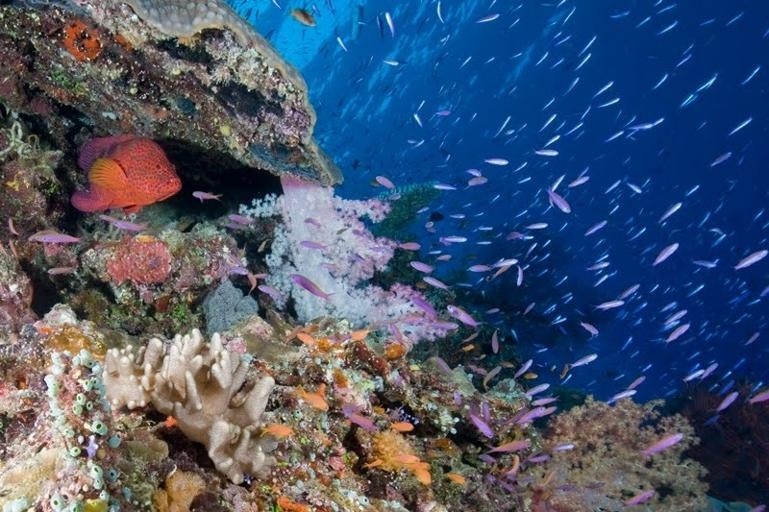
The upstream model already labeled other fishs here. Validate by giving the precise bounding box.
[219,0,769,512]
